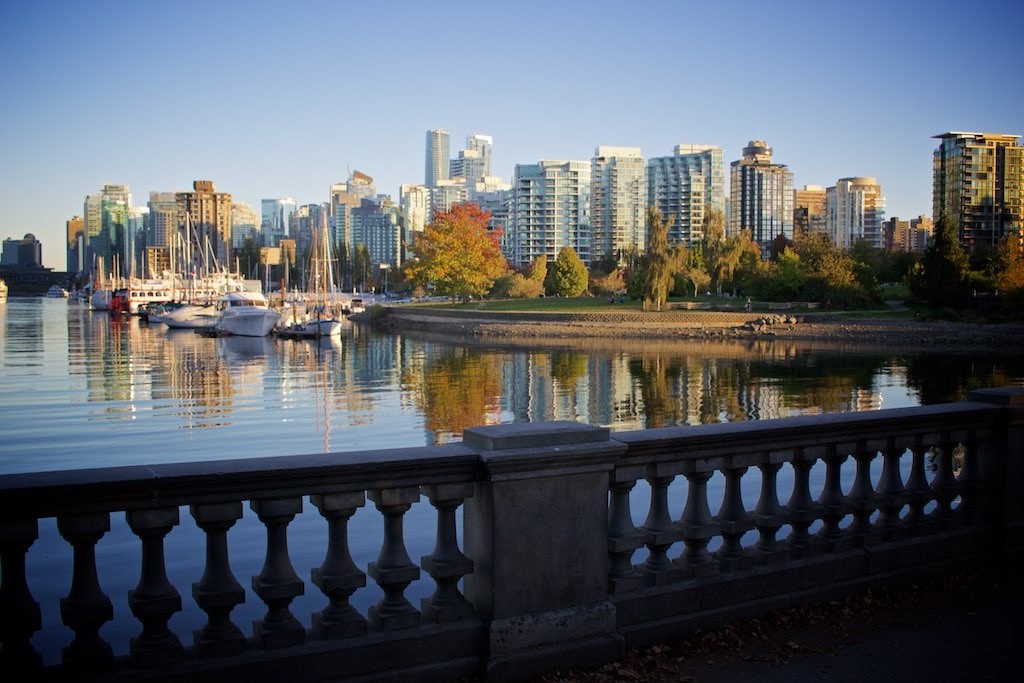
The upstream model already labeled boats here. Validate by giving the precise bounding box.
[45,284,69,297]
[0,279,8,304]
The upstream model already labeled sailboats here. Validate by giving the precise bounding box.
[86,207,367,338]
